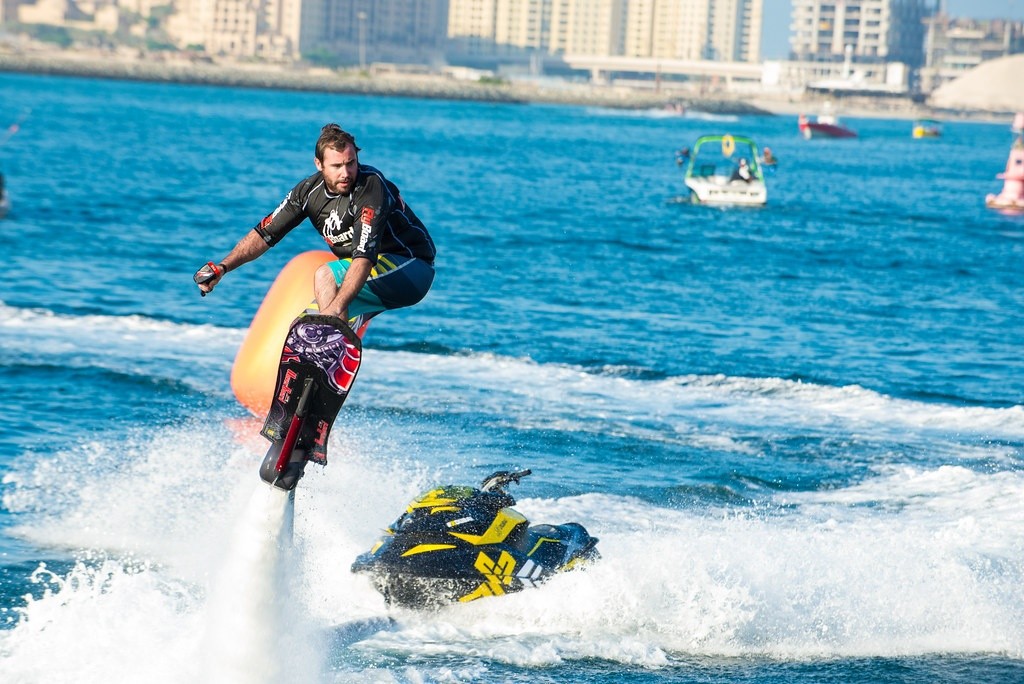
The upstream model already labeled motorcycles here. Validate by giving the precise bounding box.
[350,464,602,612]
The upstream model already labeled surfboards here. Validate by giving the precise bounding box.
[260,314,362,468]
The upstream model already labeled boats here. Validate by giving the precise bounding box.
[800,119,856,139]
[682,134,767,208]
[914,117,944,137]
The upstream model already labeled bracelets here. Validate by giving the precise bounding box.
[215,263,228,275]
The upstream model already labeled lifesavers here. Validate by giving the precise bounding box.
[720,133,736,158]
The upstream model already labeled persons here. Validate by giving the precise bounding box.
[730,157,758,181]
[762,146,775,163]
[193,122,437,336]
